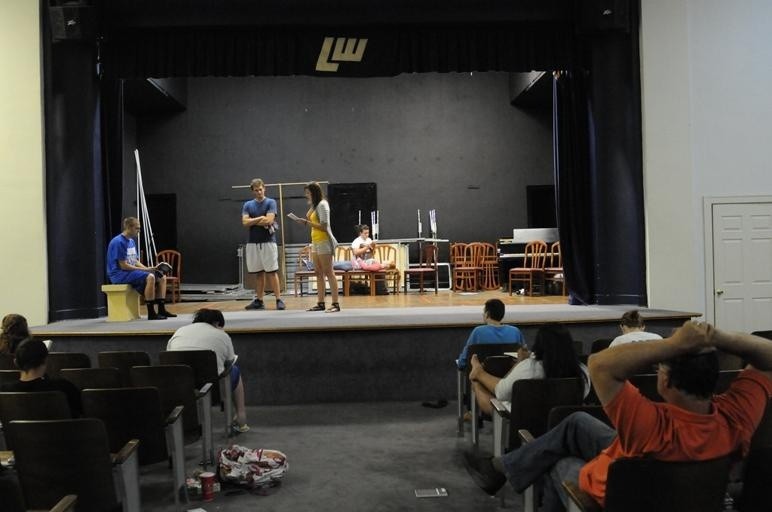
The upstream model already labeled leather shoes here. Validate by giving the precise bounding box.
[459,441,508,496]
[158,310,176,318]
[147,313,167,320]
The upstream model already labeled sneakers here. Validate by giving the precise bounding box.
[463,411,485,429]
[230,416,250,434]
[276,298,286,310]
[244,299,265,310]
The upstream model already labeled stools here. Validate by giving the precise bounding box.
[101,285,142,322]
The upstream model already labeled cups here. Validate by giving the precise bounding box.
[199,472,216,501]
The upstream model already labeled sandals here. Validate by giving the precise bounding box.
[307,301,326,312]
[325,302,340,313]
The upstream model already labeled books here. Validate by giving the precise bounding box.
[155,263,172,277]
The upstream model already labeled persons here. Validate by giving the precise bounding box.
[241,178,286,311]
[167,308,250,434]
[106,218,176,319]
[461,321,772,511]
[301,258,396,273]
[469,324,590,419]
[0,339,87,420]
[0,314,31,380]
[297,182,341,313]
[352,225,375,260]
[457,299,527,370]
[607,311,664,348]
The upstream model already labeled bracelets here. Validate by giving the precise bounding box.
[304,218,307,224]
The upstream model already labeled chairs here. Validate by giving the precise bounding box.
[579,355,588,365]
[82,387,191,512]
[9,418,141,512]
[0,353,19,371]
[0,370,57,392]
[158,349,238,438]
[0,465,79,511]
[509,241,548,297]
[0,391,76,451]
[545,240,565,296]
[713,370,772,405]
[490,377,585,458]
[326,244,346,296]
[404,245,439,295]
[471,356,517,444]
[97,351,151,369]
[582,372,665,405]
[725,445,772,512]
[733,400,772,512]
[518,405,616,512]
[59,366,122,390]
[530,340,583,356]
[346,247,372,296]
[156,250,181,304]
[454,343,522,437]
[450,242,500,292]
[130,365,214,465]
[294,245,317,297]
[561,451,741,512]
[591,338,614,354]
[373,245,399,296]
[740,330,772,371]
[46,351,92,373]
